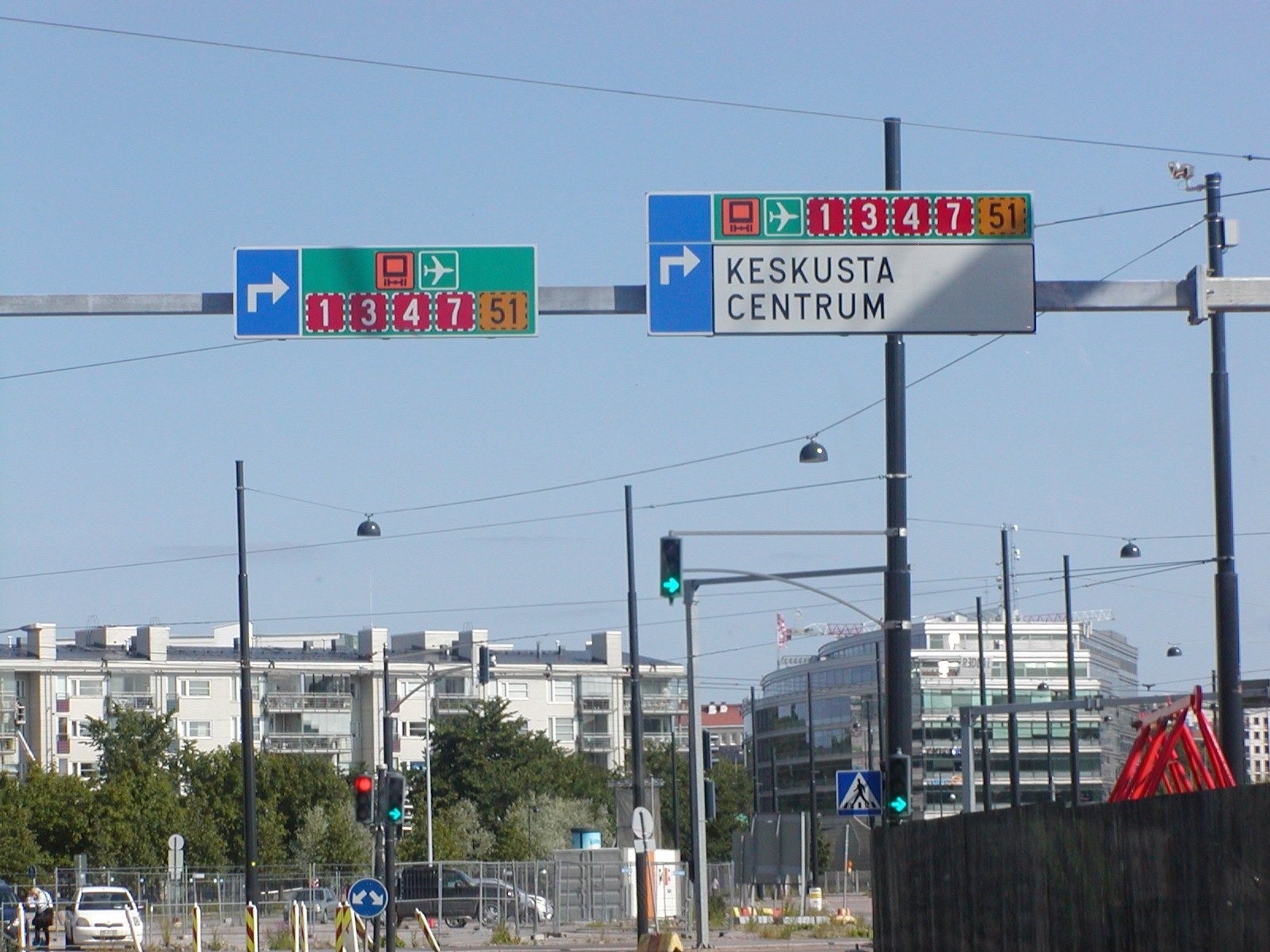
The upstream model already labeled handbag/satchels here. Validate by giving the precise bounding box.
[32,907,53,927]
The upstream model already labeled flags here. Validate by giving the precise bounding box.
[776,612,790,649]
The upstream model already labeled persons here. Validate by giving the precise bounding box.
[26,886,53,946]
[710,875,720,896]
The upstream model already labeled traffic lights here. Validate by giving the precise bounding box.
[385,771,404,825]
[659,534,683,603]
[354,773,375,826]
[883,752,917,819]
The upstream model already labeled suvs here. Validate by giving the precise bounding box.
[386,867,512,926]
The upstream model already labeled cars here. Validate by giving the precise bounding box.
[286,885,343,922]
[0,883,30,951]
[449,874,556,924]
[63,886,143,946]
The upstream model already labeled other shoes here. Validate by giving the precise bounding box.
[33,940,49,945]
